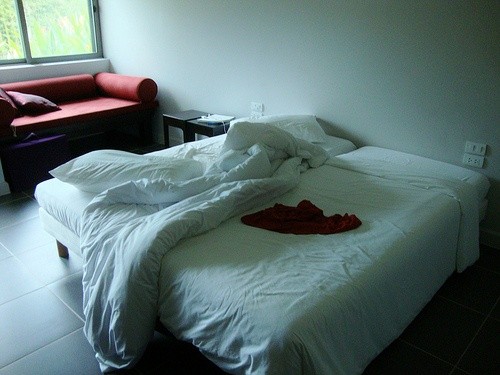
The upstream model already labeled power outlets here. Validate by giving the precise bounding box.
[462,154,486,169]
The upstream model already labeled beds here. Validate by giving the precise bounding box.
[158,147,493,375]
[33,133,357,259]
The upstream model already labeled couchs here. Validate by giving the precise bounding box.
[0,72,159,194]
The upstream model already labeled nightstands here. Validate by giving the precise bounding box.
[162,110,211,147]
[187,115,231,142]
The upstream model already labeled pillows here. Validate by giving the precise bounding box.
[47,149,204,193]
[0,88,23,117]
[5,90,62,112]
[230,113,327,142]
